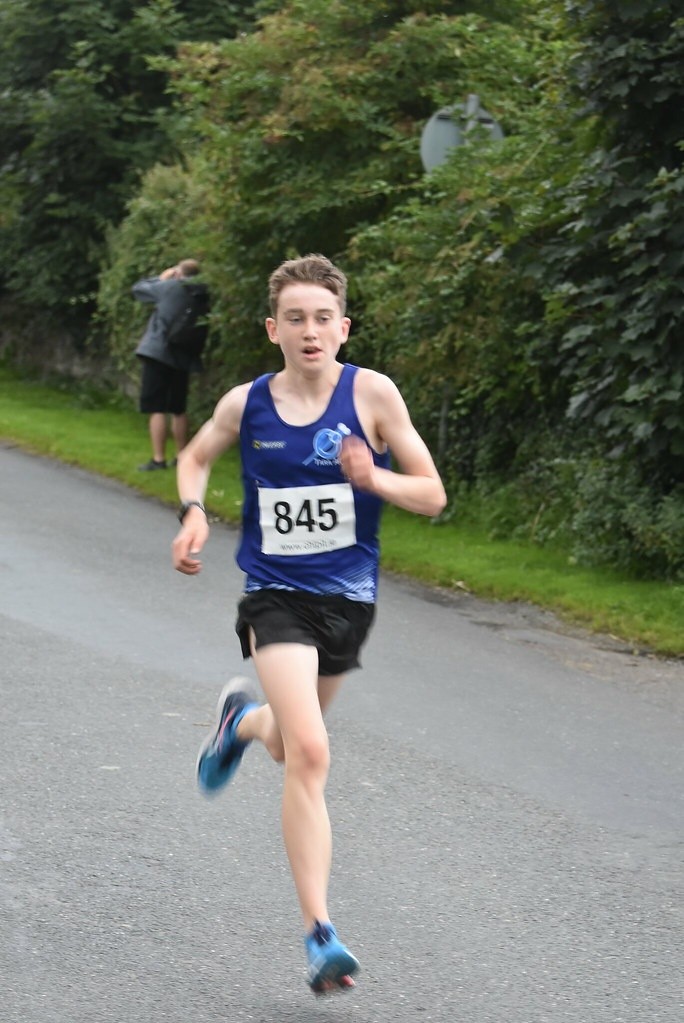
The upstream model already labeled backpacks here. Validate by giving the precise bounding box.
[160,280,210,364]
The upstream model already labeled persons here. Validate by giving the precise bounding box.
[131,257,215,474]
[169,250,448,995]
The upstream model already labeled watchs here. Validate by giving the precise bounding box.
[178,500,209,524]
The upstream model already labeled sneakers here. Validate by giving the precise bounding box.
[172,458,177,466]
[195,676,259,796]
[138,458,167,471]
[304,917,361,995]
